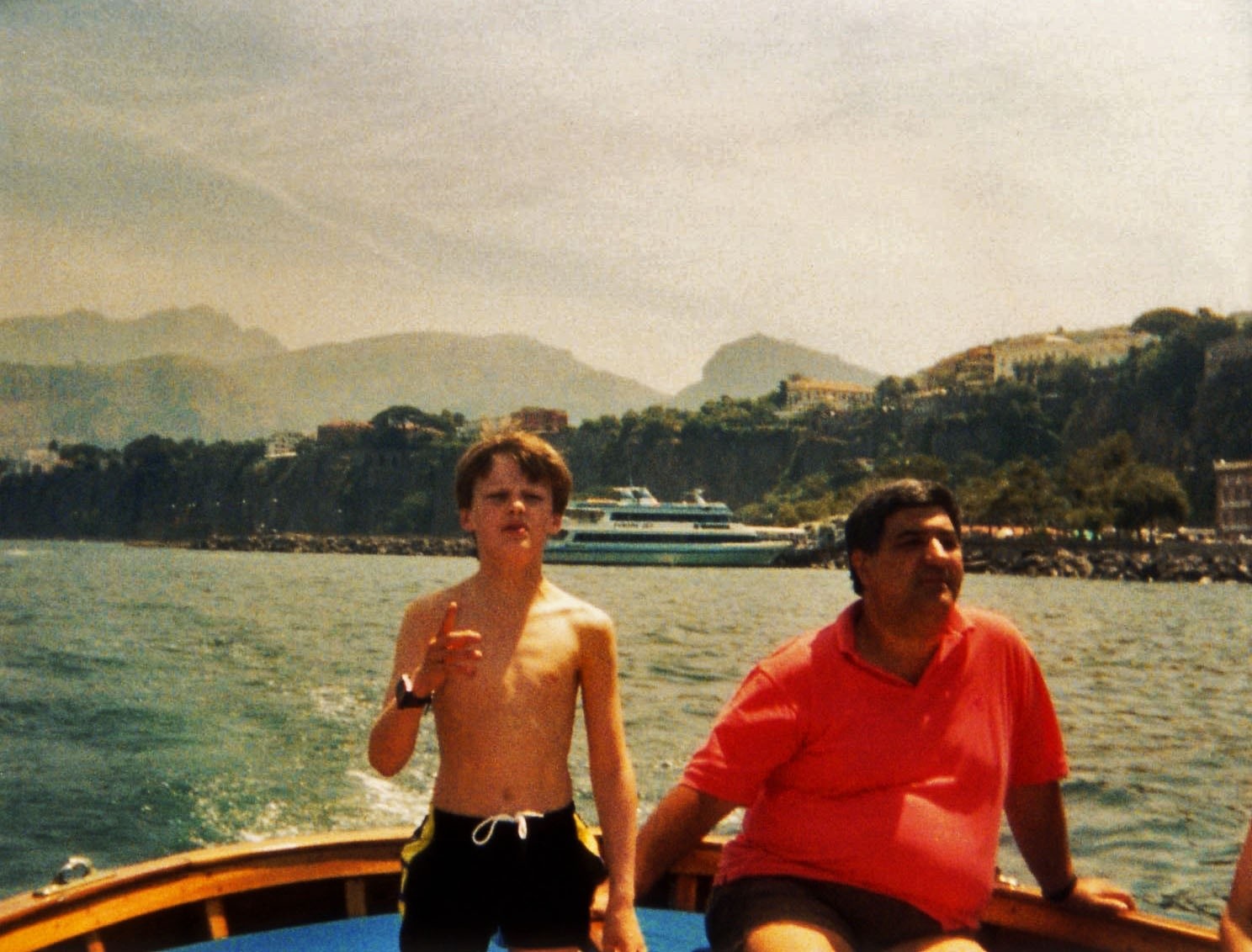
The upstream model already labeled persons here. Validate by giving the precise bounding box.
[591,476,1138,952]
[1217,820,1252,952]
[368,426,643,952]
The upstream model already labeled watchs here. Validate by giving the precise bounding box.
[396,672,435,715]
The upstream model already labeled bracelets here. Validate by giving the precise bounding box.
[1042,875,1077,903]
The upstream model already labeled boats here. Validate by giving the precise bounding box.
[539,483,810,567]
